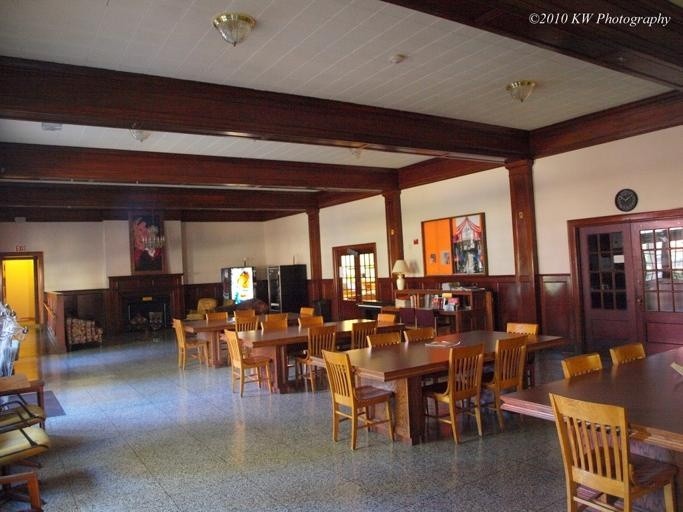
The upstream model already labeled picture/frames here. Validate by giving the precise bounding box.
[421,213,488,278]
[127,210,166,275]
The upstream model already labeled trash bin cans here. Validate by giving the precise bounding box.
[312,300,331,322]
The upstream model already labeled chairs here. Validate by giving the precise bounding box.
[609,342,646,366]
[549,392,679,512]
[0,410,51,512]
[170,307,566,451]
[0,393,46,468]
[560,351,603,378]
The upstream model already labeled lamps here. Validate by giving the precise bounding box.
[212,12,256,47]
[505,80,537,103]
[391,259,411,291]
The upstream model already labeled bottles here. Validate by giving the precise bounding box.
[442,297,448,311]
[233,269,250,304]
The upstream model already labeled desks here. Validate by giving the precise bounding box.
[499,347,683,512]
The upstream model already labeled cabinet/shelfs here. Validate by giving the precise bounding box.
[380,289,496,333]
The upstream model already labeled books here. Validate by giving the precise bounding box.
[409,293,462,311]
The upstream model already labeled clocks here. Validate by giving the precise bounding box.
[615,189,638,211]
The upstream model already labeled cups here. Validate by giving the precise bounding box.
[454,305,458,311]
[450,304,454,311]
